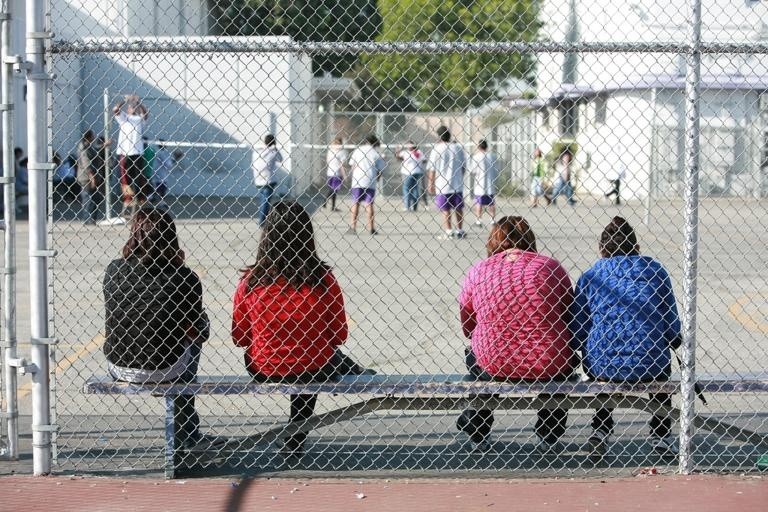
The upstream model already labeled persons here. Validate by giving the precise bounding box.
[528,146,550,209]
[453,215,582,454]
[551,146,577,206]
[51,93,172,226]
[391,125,498,241]
[248,134,283,229]
[571,216,682,462]
[229,197,376,458]
[13,146,28,216]
[321,136,348,213]
[102,207,210,449]
[343,134,388,237]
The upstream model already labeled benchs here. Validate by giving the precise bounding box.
[79,374,768,482]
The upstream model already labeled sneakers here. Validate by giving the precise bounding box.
[536,435,555,449]
[436,231,466,239]
[345,229,377,235]
[471,435,491,451]
[276,438,297,464]
[472,220,495,226]
[181,433,228,453]
[652,438,679,454]
[589,429,607,455]
